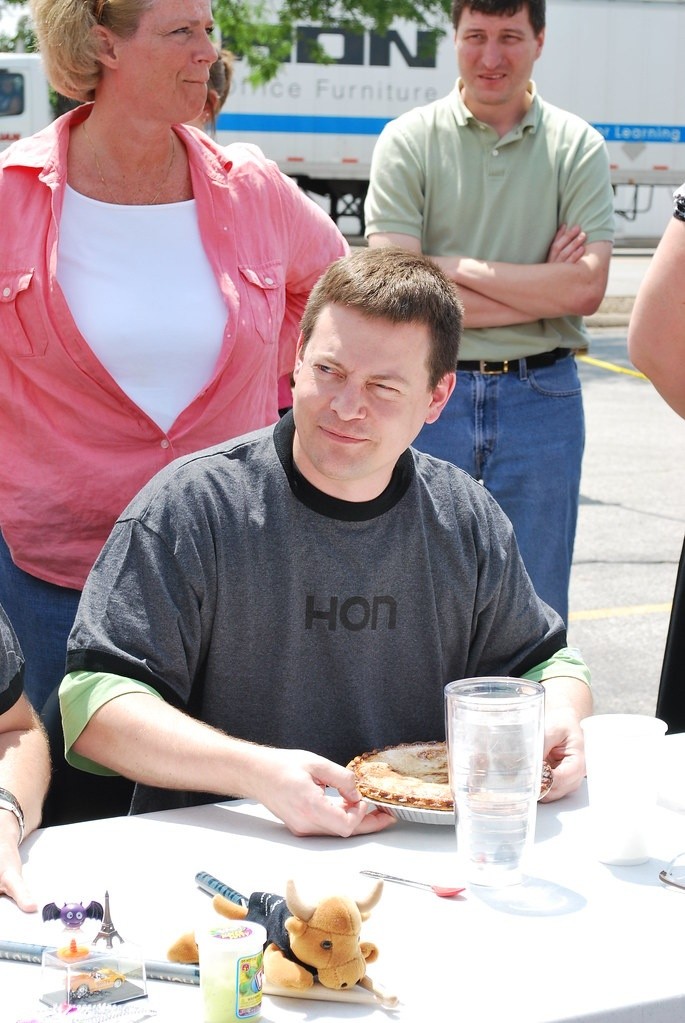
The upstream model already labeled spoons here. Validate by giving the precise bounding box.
[361,865,466,896]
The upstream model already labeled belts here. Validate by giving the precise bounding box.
[458,348,573,375]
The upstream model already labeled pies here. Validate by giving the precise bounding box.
[346,739,554,812]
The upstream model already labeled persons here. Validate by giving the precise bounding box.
[182,45,232,139]
[58,250,591,839]
[363,0,611,651]
[0,595,52,914]
[0,0,350,832]
[628,178,685,733]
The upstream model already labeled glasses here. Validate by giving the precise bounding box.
[659,853,685,890]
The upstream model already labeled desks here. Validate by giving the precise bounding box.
[0,733,685,1022]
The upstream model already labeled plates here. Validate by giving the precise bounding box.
[359,781,555,825]
[658,853,684,889]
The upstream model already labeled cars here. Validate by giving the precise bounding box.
[66,969,124,998]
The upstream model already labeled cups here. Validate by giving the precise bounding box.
[579,714,669,866]
[444,676,545,887]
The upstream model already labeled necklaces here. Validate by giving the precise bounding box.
[83,119,176,206]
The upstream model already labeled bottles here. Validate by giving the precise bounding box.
[196,921,267,1023]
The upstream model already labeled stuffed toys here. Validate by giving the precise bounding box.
[168,878,386,993]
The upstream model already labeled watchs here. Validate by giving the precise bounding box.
[0,786,25,847]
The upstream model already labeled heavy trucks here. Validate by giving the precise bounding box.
[0,0,685,237]
